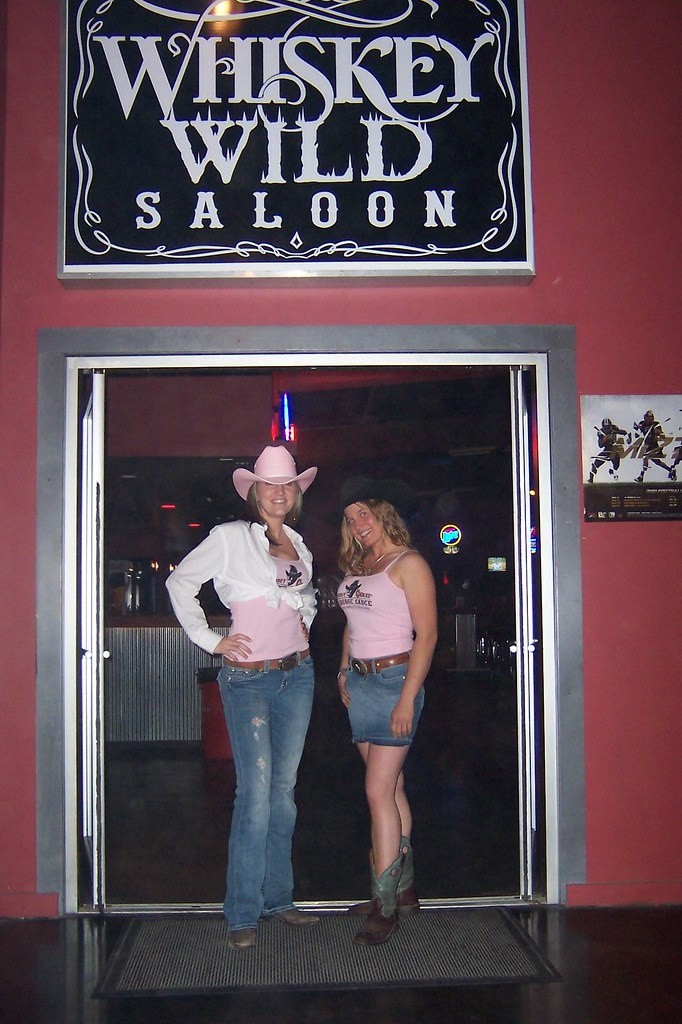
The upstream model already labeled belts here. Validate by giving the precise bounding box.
[350,650,412,675]
[218,647,313,671]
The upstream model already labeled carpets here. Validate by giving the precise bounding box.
[90,906,564,1002]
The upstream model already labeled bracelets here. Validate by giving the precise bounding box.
[337,670,344,683]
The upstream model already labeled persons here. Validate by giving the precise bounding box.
[336,472,439,947]
[165,444,319,950]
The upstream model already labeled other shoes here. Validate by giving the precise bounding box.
[230,923,257,953]
[276,903,322,927]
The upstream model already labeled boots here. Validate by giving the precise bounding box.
[352,846,405,949]
[344,837,421,918]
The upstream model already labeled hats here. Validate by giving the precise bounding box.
[329,468,410,523]
[232,444,318,501]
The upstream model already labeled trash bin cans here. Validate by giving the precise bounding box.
[197,666,232,761]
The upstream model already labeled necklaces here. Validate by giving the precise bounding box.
[363,552,388,574]
